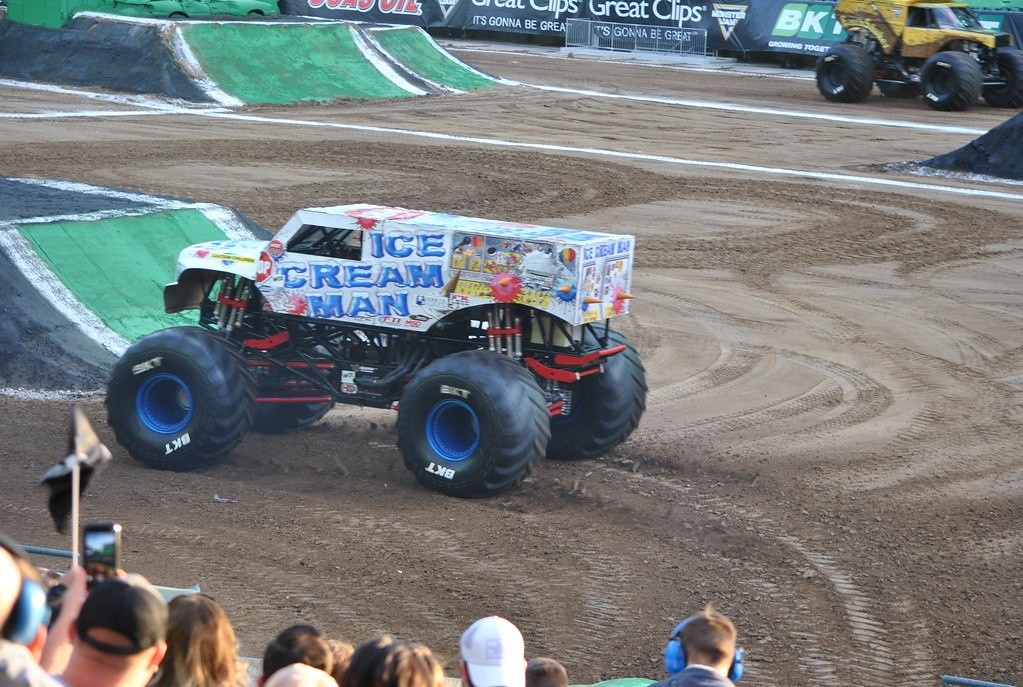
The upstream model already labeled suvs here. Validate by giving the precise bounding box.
[811,1,1023,112]
[101,203,653,499]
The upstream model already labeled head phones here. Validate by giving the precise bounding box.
[663,615,745,684]
[1,532,47,644]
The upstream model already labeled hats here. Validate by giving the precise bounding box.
[77,572,168,653]
[460,615,526,687]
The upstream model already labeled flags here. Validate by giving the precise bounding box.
[42,403,114,536]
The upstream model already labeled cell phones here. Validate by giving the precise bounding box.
[80,520,122,594]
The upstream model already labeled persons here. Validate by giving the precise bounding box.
[460,616,527,687]
[526,657,569,687]
[0,535,250,687]
[257,624,446,687]
[646,610,739,687]
